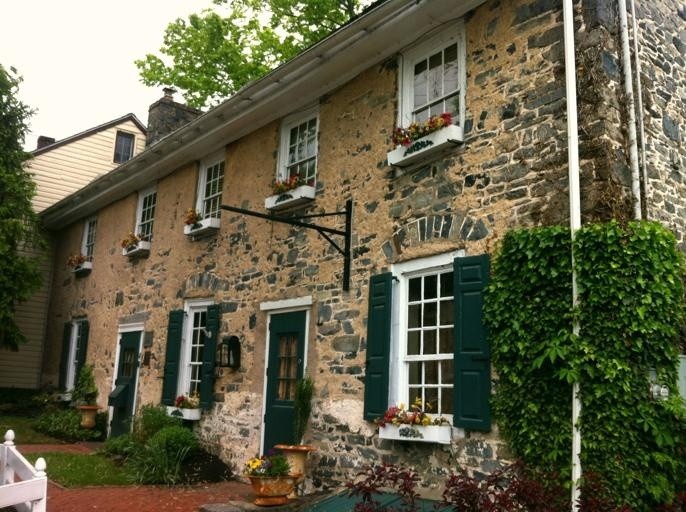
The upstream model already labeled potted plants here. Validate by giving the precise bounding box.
[69,363,105,430]
[270,373,323,494]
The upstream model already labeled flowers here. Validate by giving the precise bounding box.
[62,255,89,266]
[265,175,309,196]
[173,388,203,409]
[236,447,293,478]
[383,112,454,146]
[182,208,203,225]
[369,395,451,429]
[118,226,148,249]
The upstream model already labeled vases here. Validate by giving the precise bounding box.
[241,472,308,506]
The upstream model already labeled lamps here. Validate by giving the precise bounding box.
[210,331,243,370]
